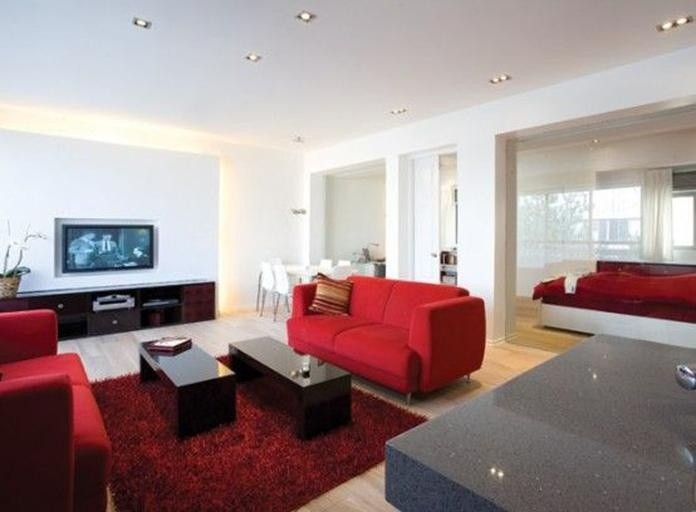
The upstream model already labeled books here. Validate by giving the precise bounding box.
[142,337,192,356]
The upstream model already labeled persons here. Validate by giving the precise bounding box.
[94,231,117,254]
[68,232,96,265]
[128,235,150,266]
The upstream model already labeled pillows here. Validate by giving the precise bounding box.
[308,271,354,317]
[530,278,566,299]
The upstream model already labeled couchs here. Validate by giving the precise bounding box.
[287,275,486,405]
[538,260,696,350]
[1,311,112,511]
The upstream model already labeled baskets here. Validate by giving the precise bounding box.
[0,267,30,297]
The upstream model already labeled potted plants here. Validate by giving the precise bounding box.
[1,224,47,302]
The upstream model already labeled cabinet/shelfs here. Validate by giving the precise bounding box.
[0,281,218,340]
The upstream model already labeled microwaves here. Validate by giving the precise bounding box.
[441,251,458,266]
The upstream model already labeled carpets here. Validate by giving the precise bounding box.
[89,343,431,510]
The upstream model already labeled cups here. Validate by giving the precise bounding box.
[298,354,310,373]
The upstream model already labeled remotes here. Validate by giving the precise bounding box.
[299,353,315,373]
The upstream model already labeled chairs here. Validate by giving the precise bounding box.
[273,257,298,320]
[257,259,290,317]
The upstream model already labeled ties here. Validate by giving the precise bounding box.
[104,241,109,252]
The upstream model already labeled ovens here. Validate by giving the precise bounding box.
[440,267,458,286]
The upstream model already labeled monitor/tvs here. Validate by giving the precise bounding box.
[54,218,160,277]
